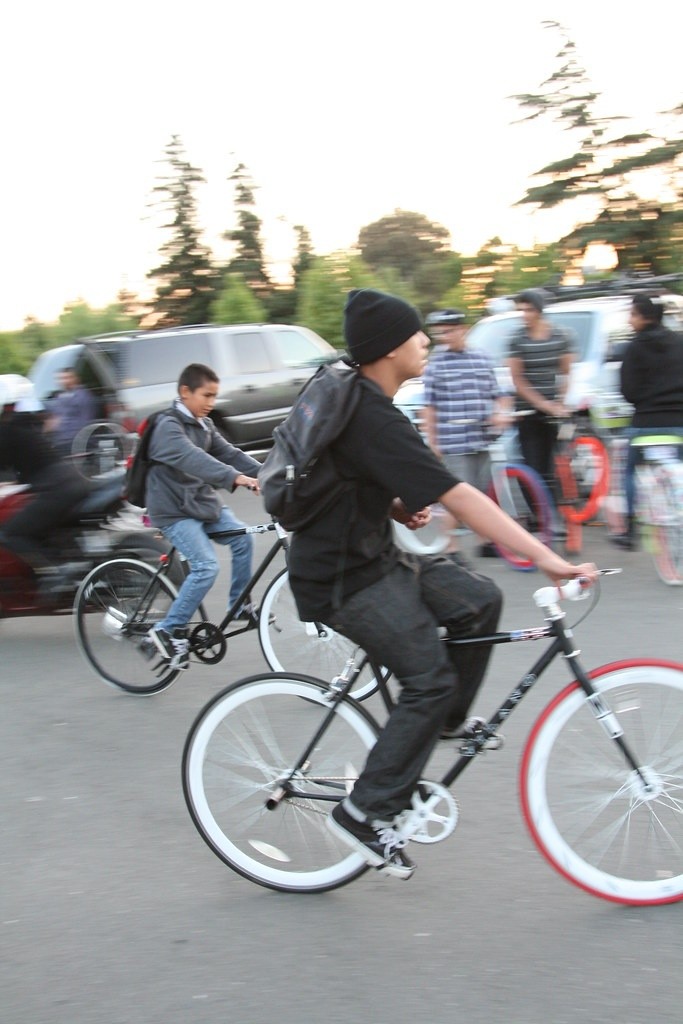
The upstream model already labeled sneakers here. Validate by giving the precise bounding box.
[147,624,192,673]
[436,716,502,750]
[324,803,413,882]
[230,601,275,625]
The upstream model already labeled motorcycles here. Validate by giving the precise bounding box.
[0,453,190,617]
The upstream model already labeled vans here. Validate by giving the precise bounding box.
[29,324,351,466]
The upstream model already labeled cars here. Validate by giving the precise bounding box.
[391,295,683,445]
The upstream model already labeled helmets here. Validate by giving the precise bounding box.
[0,373,35,406]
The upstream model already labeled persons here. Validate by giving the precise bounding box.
[144,363,276,672]
[288,288,603,879]
[0,346,118,611]
[424,307,508,558]
[608,293,683,551]
[506,288,573,544]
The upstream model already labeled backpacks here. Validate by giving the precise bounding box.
[253,358,383,529]
[120,408,192,508]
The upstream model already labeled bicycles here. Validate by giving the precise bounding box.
[555,401,683,590]
[391,410,554,573]
[181,566,682,908]
[72,487,392,707]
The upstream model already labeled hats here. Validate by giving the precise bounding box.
[424,309,460,326]
[346,289,419,364]
[506,292,545,315]
